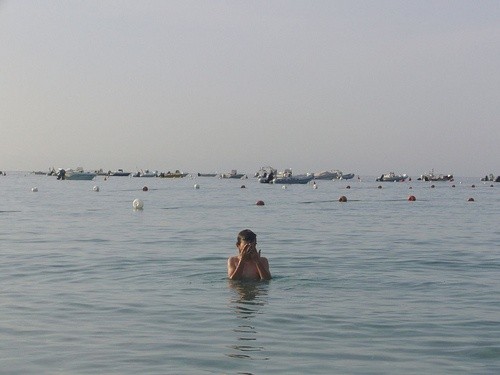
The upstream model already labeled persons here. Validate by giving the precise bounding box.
[228,230,271,281]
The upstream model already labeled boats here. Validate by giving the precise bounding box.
[220,170,248,179]
[198,172,217,177]
[63,168,189,180]
[419,168,454,182]
[376,170,411,182]
[255,168,355,183]
